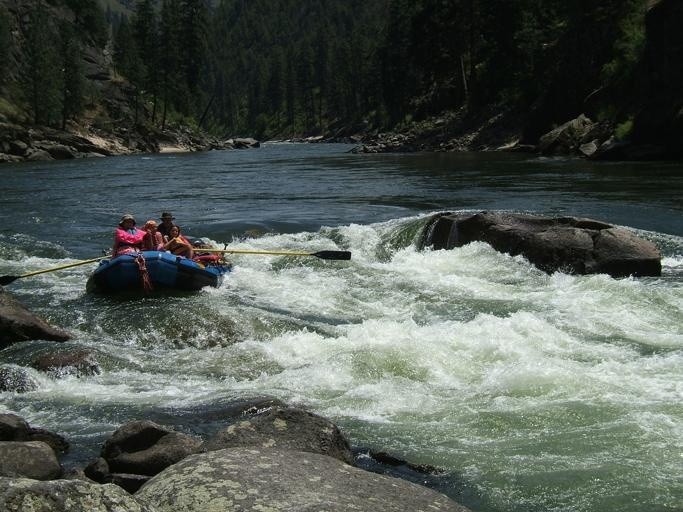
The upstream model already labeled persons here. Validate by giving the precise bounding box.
[112,212,211,263]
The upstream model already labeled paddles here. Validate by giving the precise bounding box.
[0,254,112,287]
[193,249,351,260]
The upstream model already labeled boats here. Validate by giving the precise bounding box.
[84,238,231,295]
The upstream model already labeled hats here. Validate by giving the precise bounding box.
[159,212,176,220]
[118,215,136,226]
[144,221,159,230]
